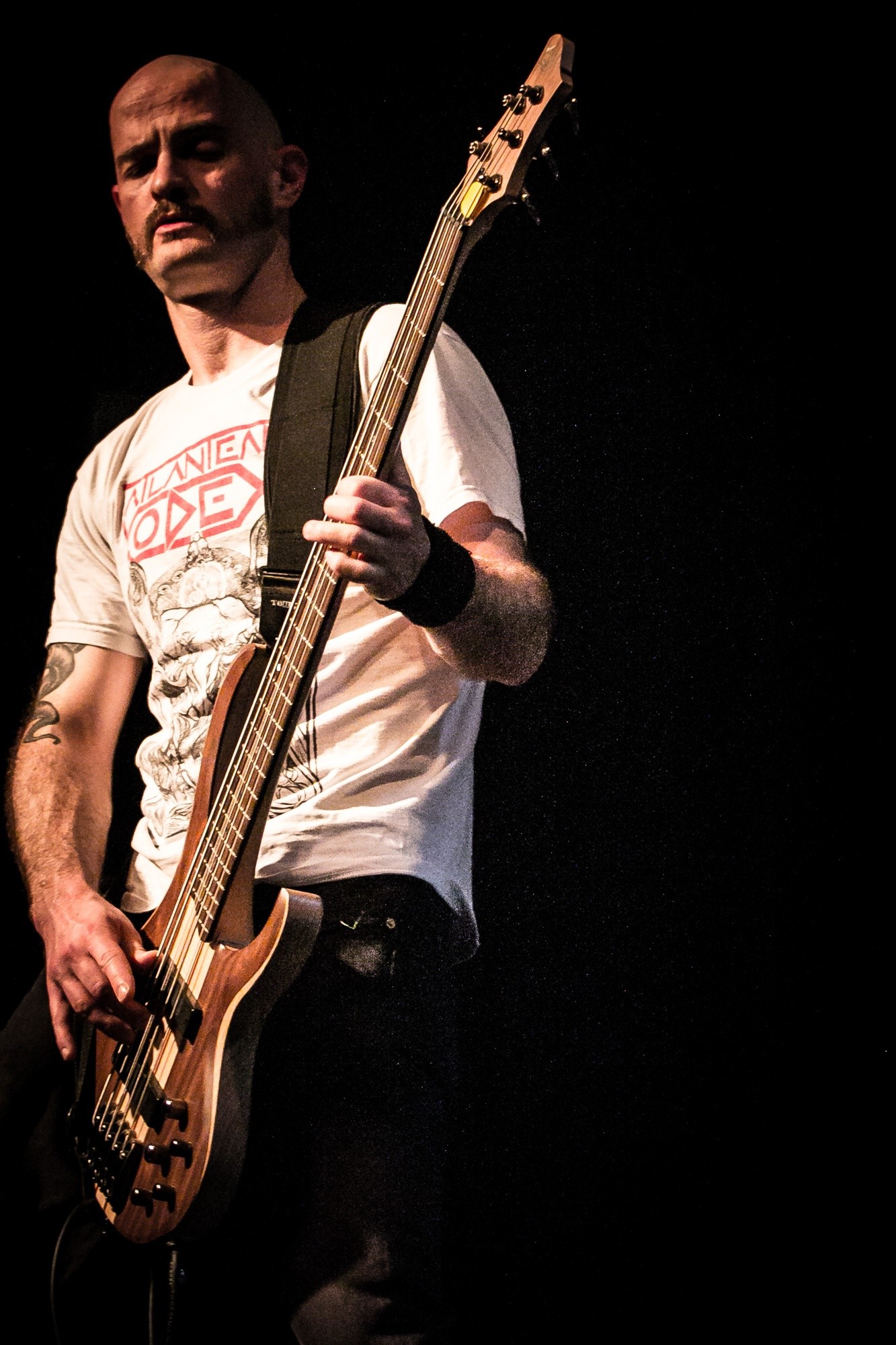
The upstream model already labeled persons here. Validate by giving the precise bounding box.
[9,55,553,1345]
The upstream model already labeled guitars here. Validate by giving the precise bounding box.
[61,27,575,1247]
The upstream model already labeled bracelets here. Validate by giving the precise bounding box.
[373,515,479,628]
